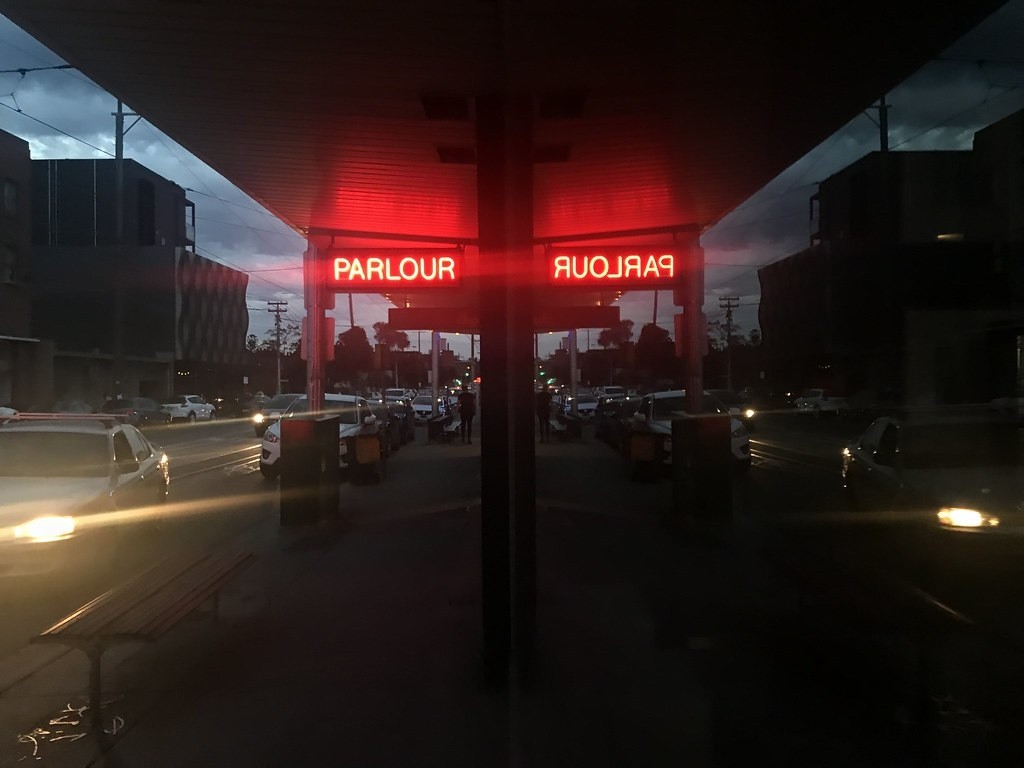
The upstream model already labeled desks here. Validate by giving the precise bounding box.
[427,414,451,442]
[551,413,583,440]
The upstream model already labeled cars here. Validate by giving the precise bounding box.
[211,385,761,477]
[159,393,218,423]
[792,388,849,421]
[839,405,1024,576]
[99,397,162,426]
[0,412,189,581]
[625,391,752,477]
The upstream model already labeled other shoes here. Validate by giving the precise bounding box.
[540,440,544,443]
[468,441,472,444]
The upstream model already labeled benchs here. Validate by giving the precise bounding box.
[30,547,268,727]
[548,418,567,438]
[443,421,461,441]
[766,535,975,727]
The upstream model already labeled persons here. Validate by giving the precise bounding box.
[458,385,475,445]
[536,386,552,444]
